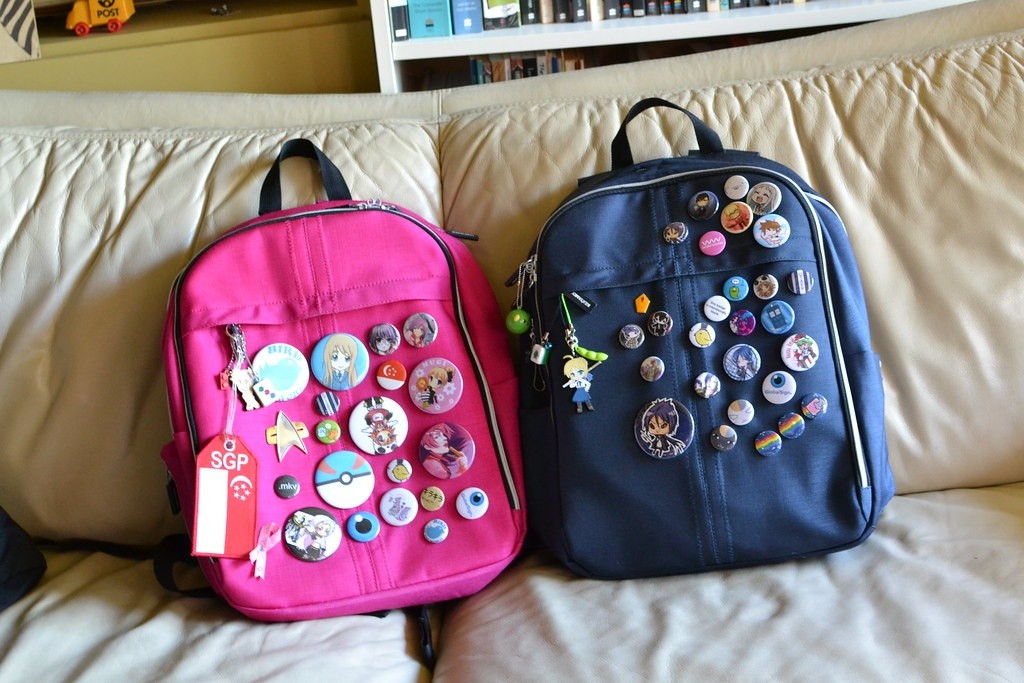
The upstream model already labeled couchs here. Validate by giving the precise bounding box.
[1,3,1023,680]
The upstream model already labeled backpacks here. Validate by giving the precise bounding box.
[504,97,894,581]
[161,139,527,622]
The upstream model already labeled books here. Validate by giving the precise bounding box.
[387,0,808,85]
